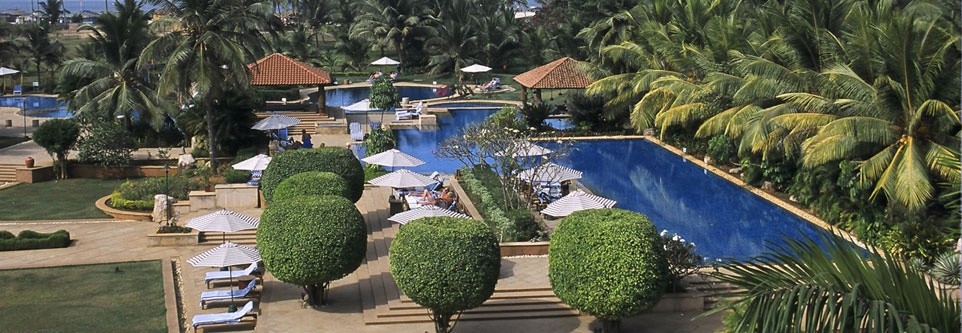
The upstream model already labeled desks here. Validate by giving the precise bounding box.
[239,276,255,290]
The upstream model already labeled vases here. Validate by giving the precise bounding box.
[26,157,35,168]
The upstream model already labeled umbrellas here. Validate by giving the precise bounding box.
[231,154,272,171]
[540,190,617,217]
[515,162,583,192]
[185,208,260,245]
[367,168,437,202]
[186,241,263,301]
[459,64,492,87]
[361,148,426,195]
[250,115,301,144]
[494,140,553,166]
[386,204,472,226]
[371,56,400,64]
[340,98,383,133]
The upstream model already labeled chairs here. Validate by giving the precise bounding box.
[201,278,263,310]
[396,100,423,120]
[205,262,262,289]
[192,300,259,333]
[397,171,459,212]
[520,180,569,219]
[13,84,22,96]
[350,122,363,141]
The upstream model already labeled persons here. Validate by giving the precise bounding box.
[479,78,496,91]
[268,129,312,148]
[369,70,397,79]
[408,187,453,209]
